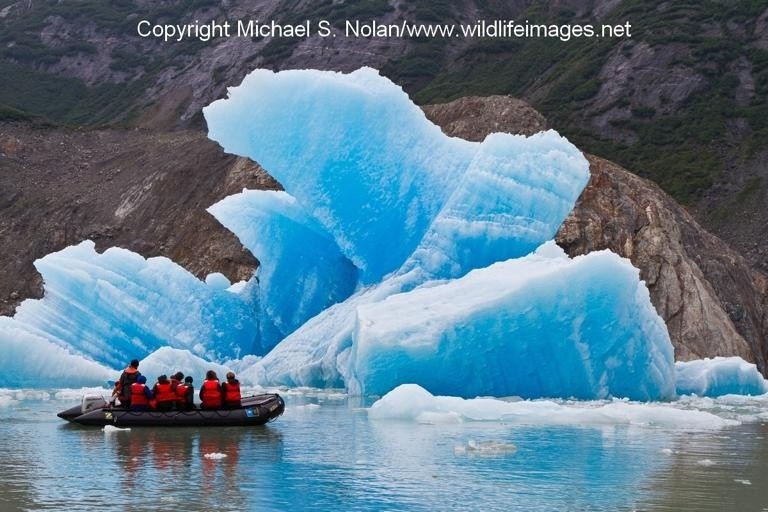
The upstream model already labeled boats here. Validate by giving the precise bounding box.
[57,392,285,427]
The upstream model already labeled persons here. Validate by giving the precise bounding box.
[112,359,197,410]
[199,370,241,408]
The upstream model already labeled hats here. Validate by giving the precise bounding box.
[132,359,139,366]
[185,377,193,383]
[227,372,236,378]
[137,376,146,383]
